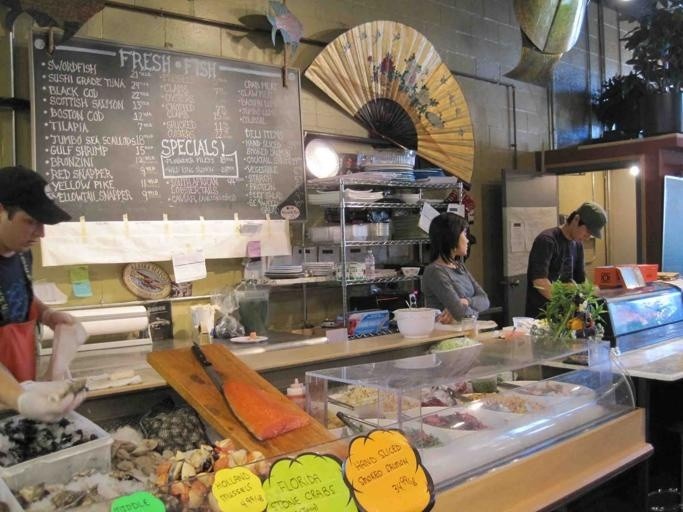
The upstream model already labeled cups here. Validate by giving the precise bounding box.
[501,326,514,339]
[460,318,479,341]
[511,333,533,361]
[511,316,534,334]
[326,328,350,344]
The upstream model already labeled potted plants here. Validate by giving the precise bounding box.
[591,2,683,138]
[534,274,608,342]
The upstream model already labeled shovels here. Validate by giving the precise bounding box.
[192,342,312,441]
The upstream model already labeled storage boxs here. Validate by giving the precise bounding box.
[0,411,114,492]
[592,264,659,289]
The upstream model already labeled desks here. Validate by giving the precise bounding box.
[539,336,683,511]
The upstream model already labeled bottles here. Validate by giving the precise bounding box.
[288,312,347,336]
[364,249,376,280]
[286,376,306,413]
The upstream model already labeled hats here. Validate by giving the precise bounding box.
[579,203,606,239]
[0,166,72,225]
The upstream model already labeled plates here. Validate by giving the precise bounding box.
[230,335,269,344]
[307,149,448,206]
[122,262,173,300]
[308,221,391,244]
[305,139,342,180]
[263,261,334,279]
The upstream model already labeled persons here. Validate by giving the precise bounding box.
[522,202,607,316]
[419,215,493,324]
[0,164,92,427]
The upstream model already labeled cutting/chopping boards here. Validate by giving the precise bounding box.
[144,341,353,479]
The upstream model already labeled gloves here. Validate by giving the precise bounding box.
[44,318,88,380]
[18,387,88,424]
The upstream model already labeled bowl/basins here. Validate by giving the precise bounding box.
[393,307,436,340]
[400,266,420,279]
[306,365,596,483]
[396,353,436,369]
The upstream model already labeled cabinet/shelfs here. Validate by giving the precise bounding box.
[305,335,654,511]
[302,179,467,326]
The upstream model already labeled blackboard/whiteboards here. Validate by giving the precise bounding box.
[29,27,309,221]
